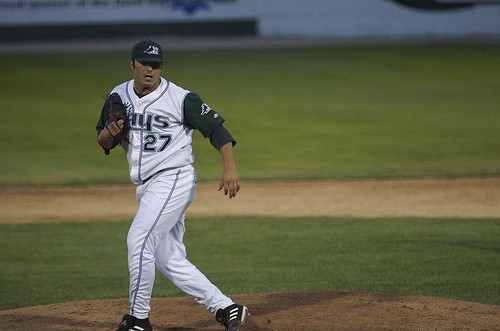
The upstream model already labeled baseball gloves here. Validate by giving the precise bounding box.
[104,93,128,141]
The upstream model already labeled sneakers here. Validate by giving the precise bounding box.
[114,314,153,331]
[220,304,248,331]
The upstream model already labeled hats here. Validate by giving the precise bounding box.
[130,41,163,63]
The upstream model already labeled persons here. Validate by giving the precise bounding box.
[96,41,247,331]
